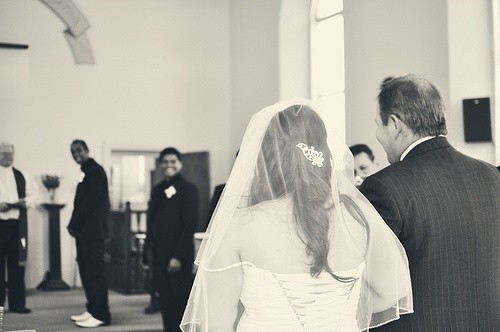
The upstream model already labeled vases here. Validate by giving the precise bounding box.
[49,187,55,203]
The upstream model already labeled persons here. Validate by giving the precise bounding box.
[179,98,414,332]
[67,140,112,329]
[358,72,500,332]
[346,143,379,188]
[0,140,32,313]
[140,147,240,332]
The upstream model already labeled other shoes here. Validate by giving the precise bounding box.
[9,307,30,314]
[75,316,106,328]
[145,305,160,314]
[71,311,92,321]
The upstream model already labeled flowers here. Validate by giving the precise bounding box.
[163,185,177,199]
[42,174,61,189]
